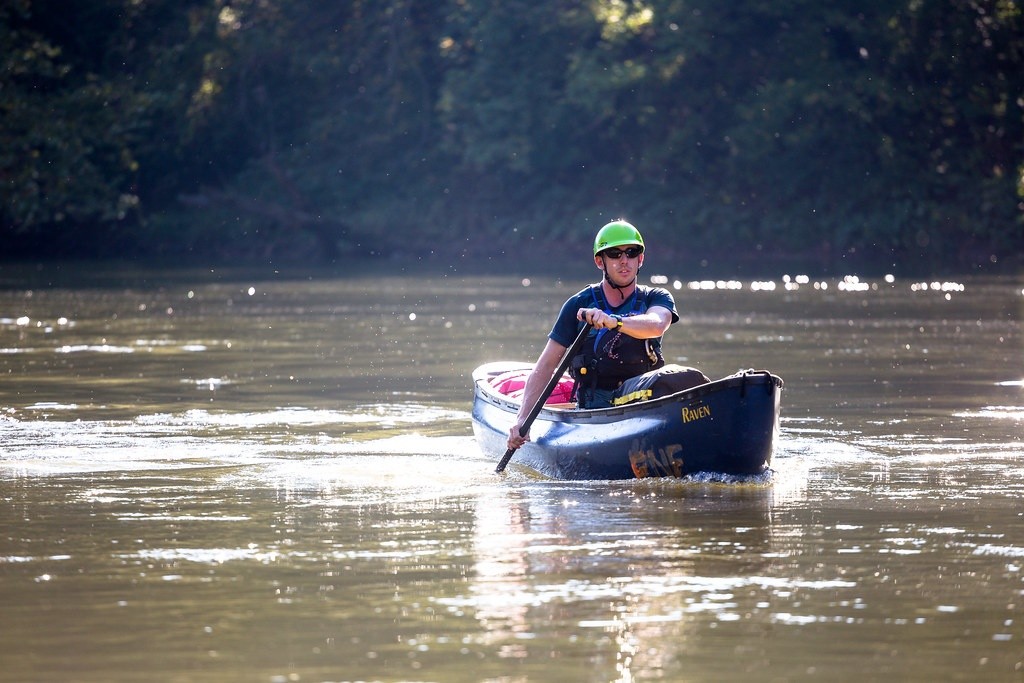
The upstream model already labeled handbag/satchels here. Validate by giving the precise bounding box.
[568,354,614,408]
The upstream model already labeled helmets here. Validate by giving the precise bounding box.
[593,222,645,256]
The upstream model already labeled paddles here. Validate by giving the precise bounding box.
[495,312,606,473]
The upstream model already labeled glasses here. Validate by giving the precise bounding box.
[606,247,642,259]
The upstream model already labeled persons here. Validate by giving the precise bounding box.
[508,220,681,451]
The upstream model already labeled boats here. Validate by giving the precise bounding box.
[471,361,783,485]
